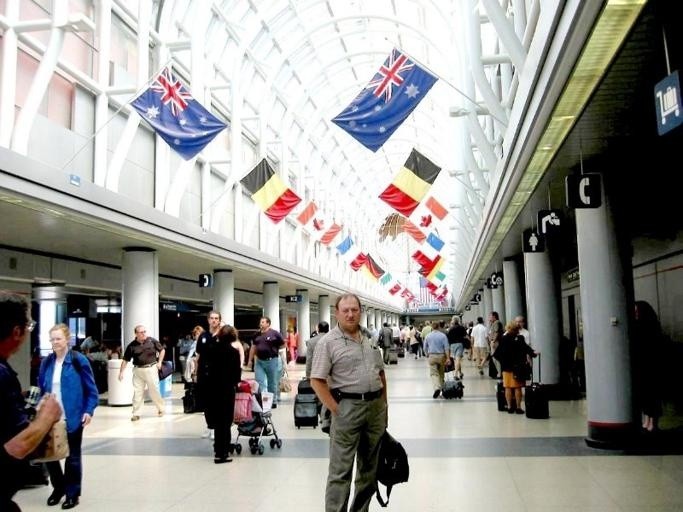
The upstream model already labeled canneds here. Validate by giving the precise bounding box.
[24,385,41,405]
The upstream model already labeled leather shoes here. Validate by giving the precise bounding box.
[61,495,79,509]
[46,484,65,506]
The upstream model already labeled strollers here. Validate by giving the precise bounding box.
[227,381,280,455]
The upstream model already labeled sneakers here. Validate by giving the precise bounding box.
[507,406,524,414]
[131,408,166,421]
[433,389,441,399]
[200,427,235,464]
[453,373,464,379]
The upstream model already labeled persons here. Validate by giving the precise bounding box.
[34,322,100,510]
[633,300,664,435]
[0,287,65,511]
[245,316,289,409]
[117,324,166,421]
[76,325,254,382]
[196,323,242,465]
[305,291,389,511]
[194,309,223,440]
[288,311,585,422]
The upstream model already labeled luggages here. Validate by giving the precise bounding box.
[388,347,405,365]
[443,380,464,399]
[497,378,517,411]
[524,382,550,419]
[294,380,319,430]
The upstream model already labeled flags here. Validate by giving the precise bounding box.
[128,67,228,162]
[377,147,442,219]
[239,158,301,225]
[295,190,454,307]
[330,49,439,153]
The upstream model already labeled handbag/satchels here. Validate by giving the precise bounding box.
[379,430,411,486]
[157,358,174,381]
[182,384,204,414]
[444,357,456,372]
